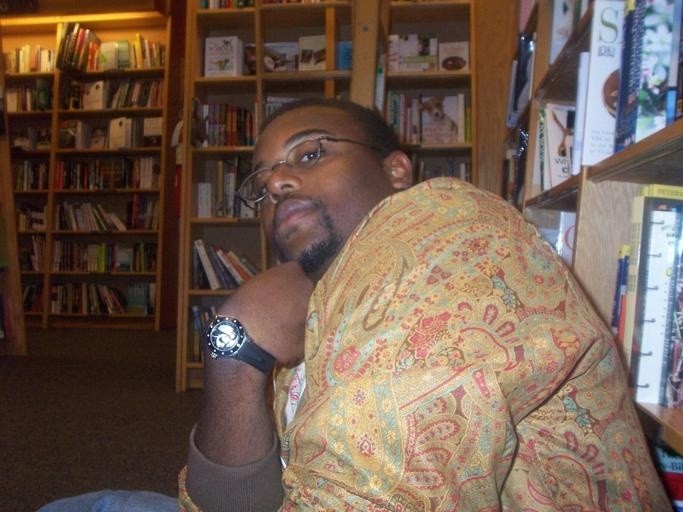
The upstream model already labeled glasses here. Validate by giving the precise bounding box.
[234,136,386,212]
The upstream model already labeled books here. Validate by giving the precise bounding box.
[5,21,165,315]
[374,33,472,185]
[195,99,287,289]
[496,2,681,505]
[205,0,353,71]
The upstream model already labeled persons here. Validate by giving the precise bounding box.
[38,99,673,511]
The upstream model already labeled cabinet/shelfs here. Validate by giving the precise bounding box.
[1,2,180,332]
[179,1,477,387]
[506,1,682,507]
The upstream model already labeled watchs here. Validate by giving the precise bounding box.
[206,312,277,379]
